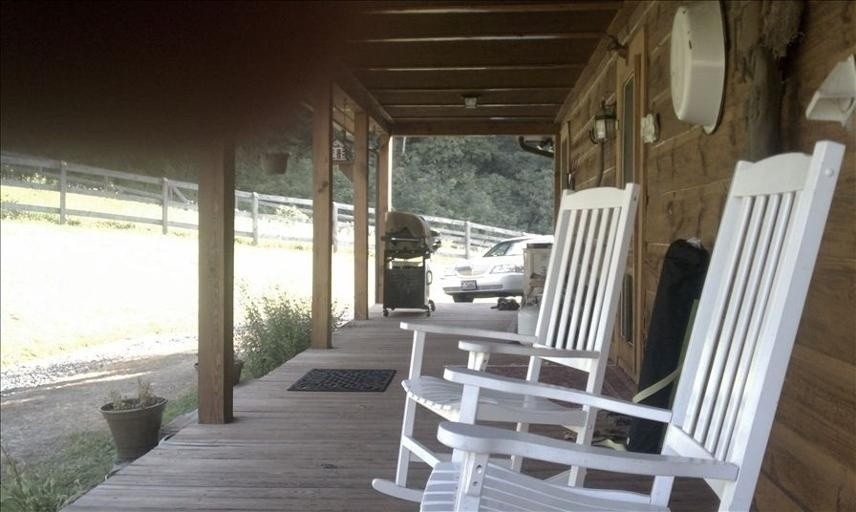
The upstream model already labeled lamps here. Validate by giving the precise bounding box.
[593,99,616,143]
[462,95,479,109]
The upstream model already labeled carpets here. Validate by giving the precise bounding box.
[286,370,396,393]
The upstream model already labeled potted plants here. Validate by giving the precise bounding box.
[250,126,298,175]
[194,344,244,386]
[100,378,167,461]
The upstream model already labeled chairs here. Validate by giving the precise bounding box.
[420,138,847,512]
[372,182,639,505]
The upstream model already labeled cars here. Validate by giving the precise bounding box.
[441,235,553,304]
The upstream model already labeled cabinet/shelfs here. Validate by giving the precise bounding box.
[523,249,551,303]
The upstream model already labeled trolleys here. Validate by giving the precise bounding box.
[380,211,440,316]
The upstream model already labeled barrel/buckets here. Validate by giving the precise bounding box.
[517,305,539,347]
